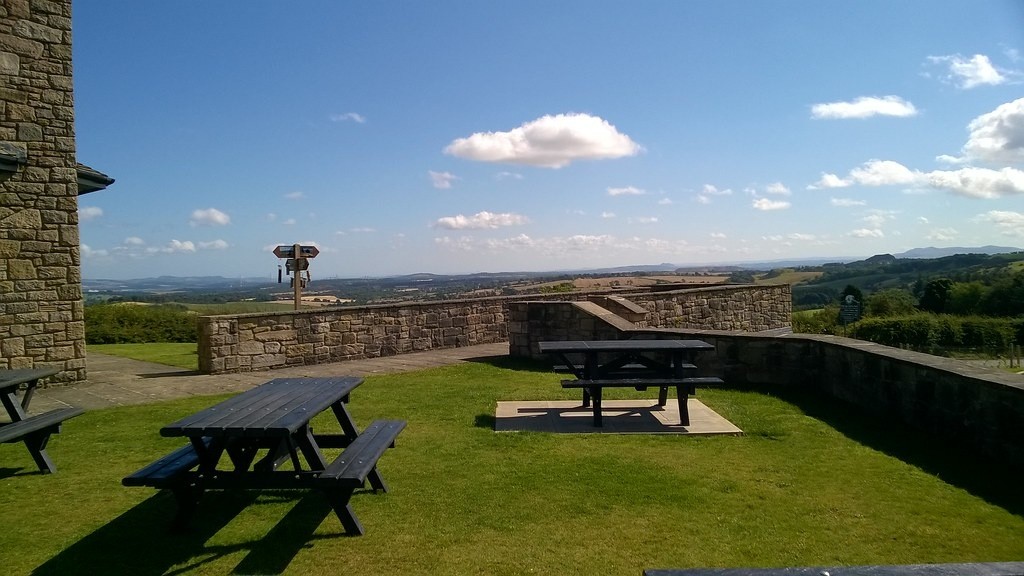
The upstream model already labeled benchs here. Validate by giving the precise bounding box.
[553,361,697,375]
[121,435,218,489]
[560,378,725,389]
[0,407,87,444]
[320,414,409,489]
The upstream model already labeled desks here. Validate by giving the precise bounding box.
[158,377,389,539]
[0,366,64,474]
[537,339,715,428]
